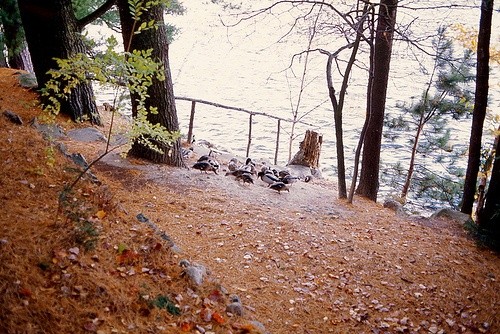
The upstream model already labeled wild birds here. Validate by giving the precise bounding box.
[182,147,301,194]
